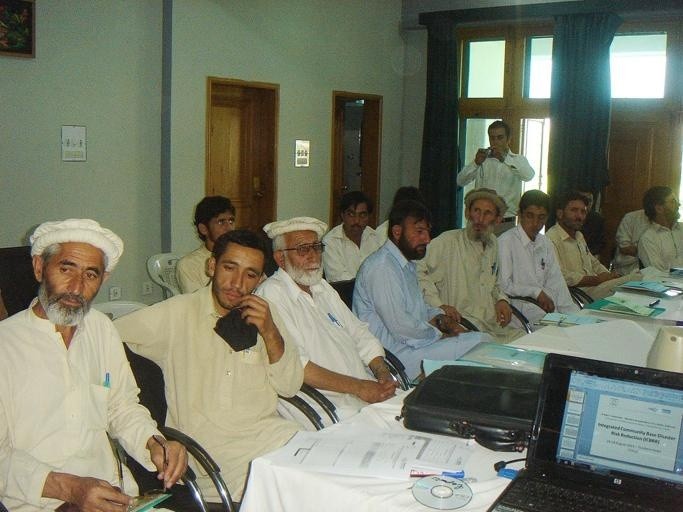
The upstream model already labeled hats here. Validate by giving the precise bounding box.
[28,217,124,275]
[260,216,328,240]
[463,187,508,217]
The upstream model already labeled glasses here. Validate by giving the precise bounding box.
[273,242,326,255]
[115,433,171,503]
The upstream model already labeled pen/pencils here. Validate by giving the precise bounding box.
[586,246,588,255]
[649,299,660,306]
[410,471,465,478]
[541,258,545,270]
[327,313,343,328]
[491,262,496,275]
[103,373,110,388]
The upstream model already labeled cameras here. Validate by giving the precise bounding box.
[483,148,492,157]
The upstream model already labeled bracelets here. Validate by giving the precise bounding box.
[500,152,507,163]
[372,365,390,376]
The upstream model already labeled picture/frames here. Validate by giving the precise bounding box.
[0,0,36,58]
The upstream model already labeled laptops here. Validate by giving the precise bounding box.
[486,352,683,512]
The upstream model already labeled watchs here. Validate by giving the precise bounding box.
[436,312,444,330]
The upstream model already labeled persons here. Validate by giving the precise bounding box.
[352,202,479,382]
[611,189,652,275]
[637,187,683,271]
[112,230,305,511]
[579,191,611,271]
[174,192,237,294]
[376,184,421,238]
[455,120,534,237]
[546,192,641,306]
[321,193,379,285]
[253,217,405,426]
[414,187,527,345]
[0,218,189,512]
[496,189,575,328]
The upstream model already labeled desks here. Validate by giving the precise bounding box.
[232,269,683,512]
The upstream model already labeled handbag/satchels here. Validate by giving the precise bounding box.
[398,362,544,453]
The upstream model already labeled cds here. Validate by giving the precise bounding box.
[410,474,473,510]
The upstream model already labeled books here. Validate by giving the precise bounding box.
[422,265,683,383]
[540,309,607,330]
